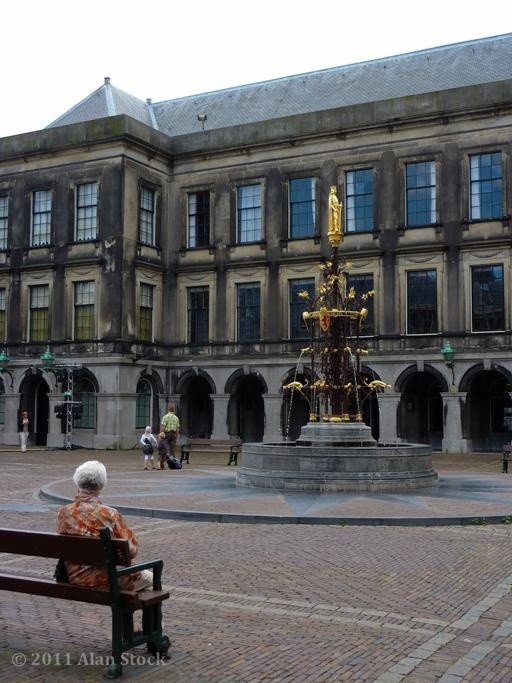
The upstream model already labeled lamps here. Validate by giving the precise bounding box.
[40,351,59,389]
[440,340,457,386]
[0,350,15,388]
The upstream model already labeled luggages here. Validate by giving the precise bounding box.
[166,454,182,469]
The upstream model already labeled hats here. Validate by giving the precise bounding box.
[146,426,152,431]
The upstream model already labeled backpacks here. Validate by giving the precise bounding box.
[143,438,153,455]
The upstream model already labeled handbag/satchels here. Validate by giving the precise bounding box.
[53,559,68,583]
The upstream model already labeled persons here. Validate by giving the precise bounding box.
[140,425,158,470]
[18,410,33,452]
[58,460,170,654]
[160,403,180,457]
[328,184,343,232]
[154,432,169,470]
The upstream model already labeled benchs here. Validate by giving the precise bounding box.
[499,445,512,473]
[0,525,172,681]
[179,438,243,466]
